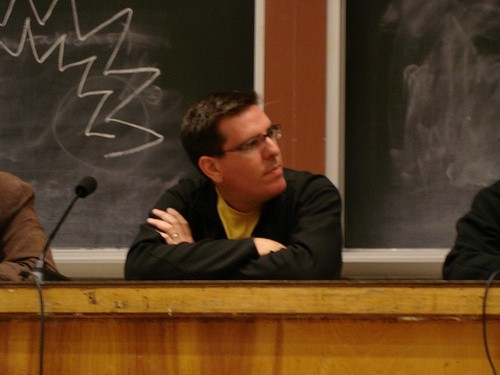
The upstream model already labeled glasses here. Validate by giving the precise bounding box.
[225,123,282,154]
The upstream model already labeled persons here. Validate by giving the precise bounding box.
[443,178,500,281]
[0,171,59,282]
[124,92,342,280]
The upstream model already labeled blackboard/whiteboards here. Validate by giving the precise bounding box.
[340,1,499,249]
[0,0,254,247]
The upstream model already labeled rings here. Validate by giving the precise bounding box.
[171,234,178,239]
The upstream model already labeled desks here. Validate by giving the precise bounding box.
[1,282,500,371]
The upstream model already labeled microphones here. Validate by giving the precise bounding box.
[33,176,98,281]
